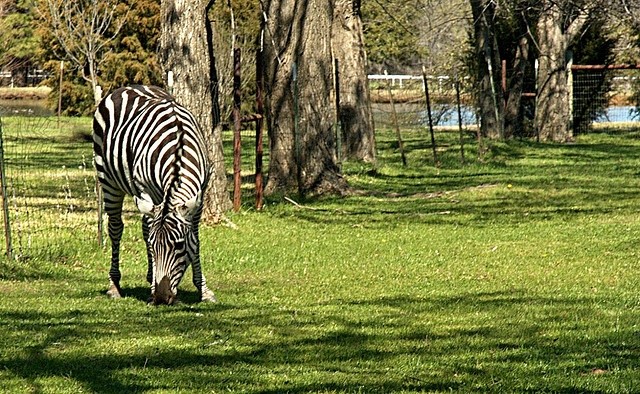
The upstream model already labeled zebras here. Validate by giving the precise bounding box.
[92,83,217,307]
